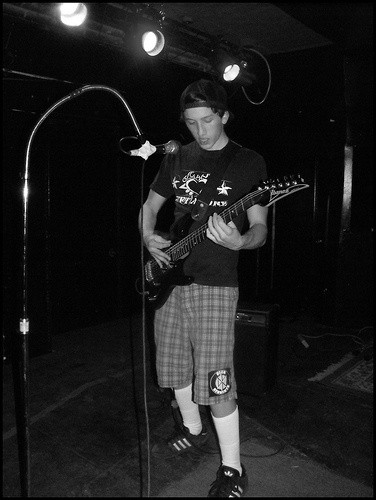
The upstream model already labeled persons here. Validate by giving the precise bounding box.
[137,78,270,500]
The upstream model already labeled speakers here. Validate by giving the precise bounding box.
[233,302,281,397]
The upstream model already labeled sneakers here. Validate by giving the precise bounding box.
[152,424,208,459]
[208,463,248,498]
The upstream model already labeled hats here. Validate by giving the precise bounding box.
[180,79,234,125]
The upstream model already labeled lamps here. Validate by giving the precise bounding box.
[208,36,241,83]
[124,5,165,58]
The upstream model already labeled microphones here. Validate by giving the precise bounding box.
[129,140,181,156]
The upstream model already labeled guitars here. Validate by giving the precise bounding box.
[124,171,309,310]
